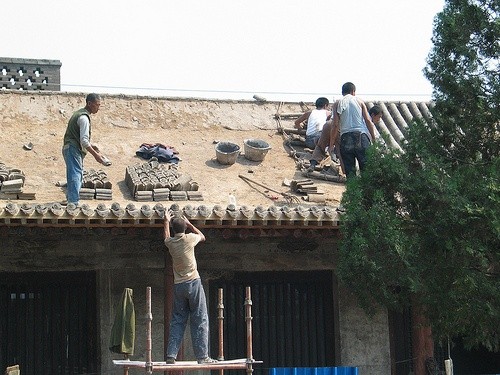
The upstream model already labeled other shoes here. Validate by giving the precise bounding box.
[310,160,317,166]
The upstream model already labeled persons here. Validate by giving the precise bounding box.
[328,82,376,190]
[307,105,383,172]
[295,97,332,150]
[62,93,105,205]
[164,207,219,364]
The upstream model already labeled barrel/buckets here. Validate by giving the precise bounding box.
[244,138,270,163]
[215,141,241,165]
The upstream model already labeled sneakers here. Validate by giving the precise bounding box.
[198,357,218,364]
[166,359,175,364]
